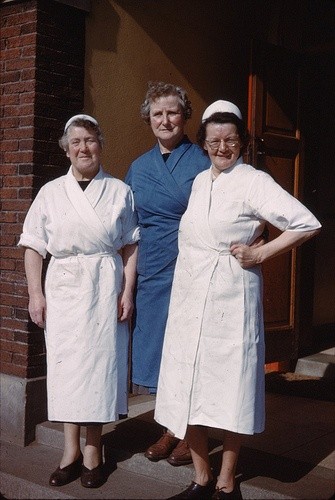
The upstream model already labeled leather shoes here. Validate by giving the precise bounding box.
[144,423,193,465]
[170,479,239,500]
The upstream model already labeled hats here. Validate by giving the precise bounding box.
[62,112,101,134]
[200,97,247,124]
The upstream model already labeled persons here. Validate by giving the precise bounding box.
[17,113,141,488]
[122,81,212,465]
[154,101,322,500]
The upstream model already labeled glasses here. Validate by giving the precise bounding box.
[205,137,244,149]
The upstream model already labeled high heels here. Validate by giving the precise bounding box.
[48,449,104,487]
